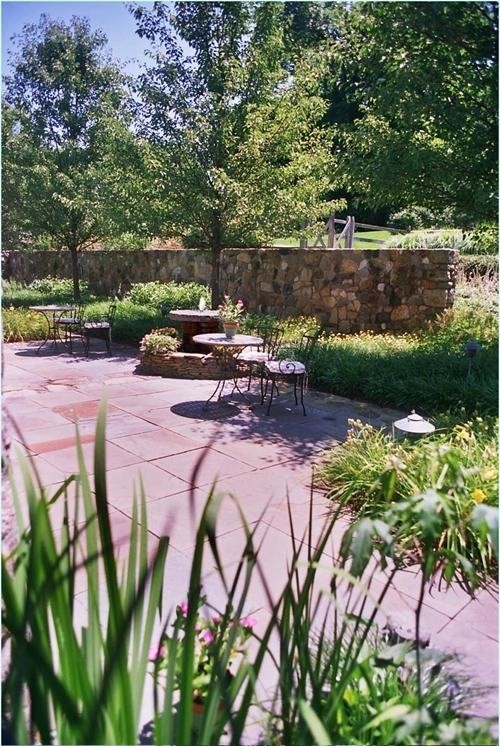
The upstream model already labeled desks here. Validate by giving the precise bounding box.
[192,332,264,412]
[28,304,75,357]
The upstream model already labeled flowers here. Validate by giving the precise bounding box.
[214,295,249,322]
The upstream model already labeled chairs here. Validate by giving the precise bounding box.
[54,302,87,351]
[83,302,118,359]
[231,327,286,406]
[261,325,321,417]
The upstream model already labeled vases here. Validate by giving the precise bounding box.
[225,322,240,338]
